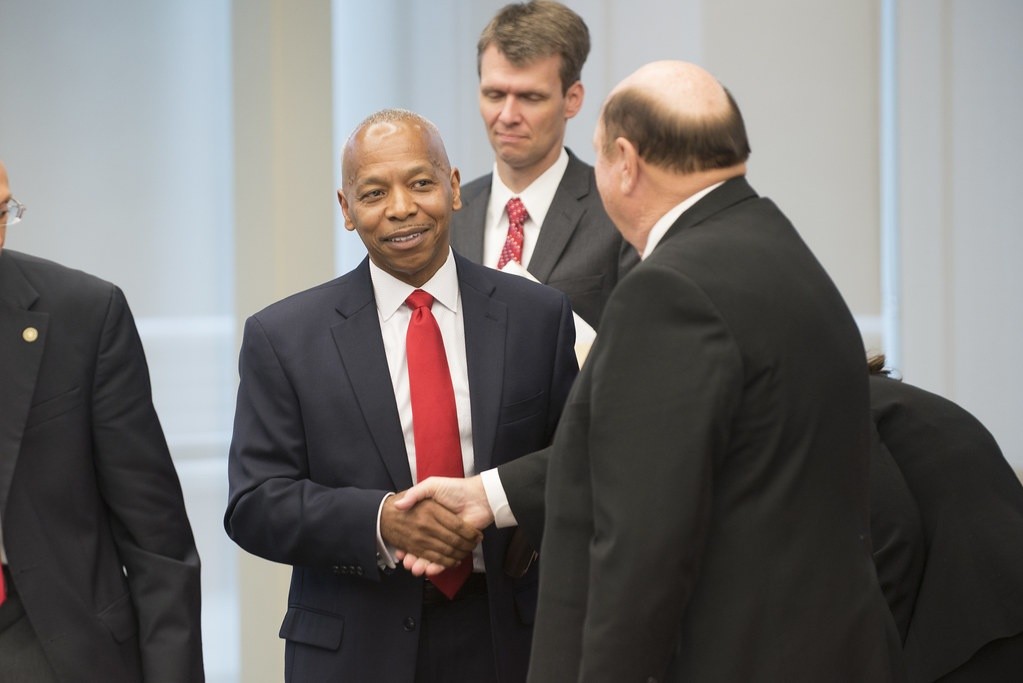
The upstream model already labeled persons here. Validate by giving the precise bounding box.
[446,0,640,370]
[223,107,579,683]
[867,356,1023,683]
[0,162,206,683]
[394,60,927,683]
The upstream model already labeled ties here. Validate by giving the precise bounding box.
[407,288,472,598]
[497,198,530,275]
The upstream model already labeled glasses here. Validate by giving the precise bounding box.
[1,197,24,228]
